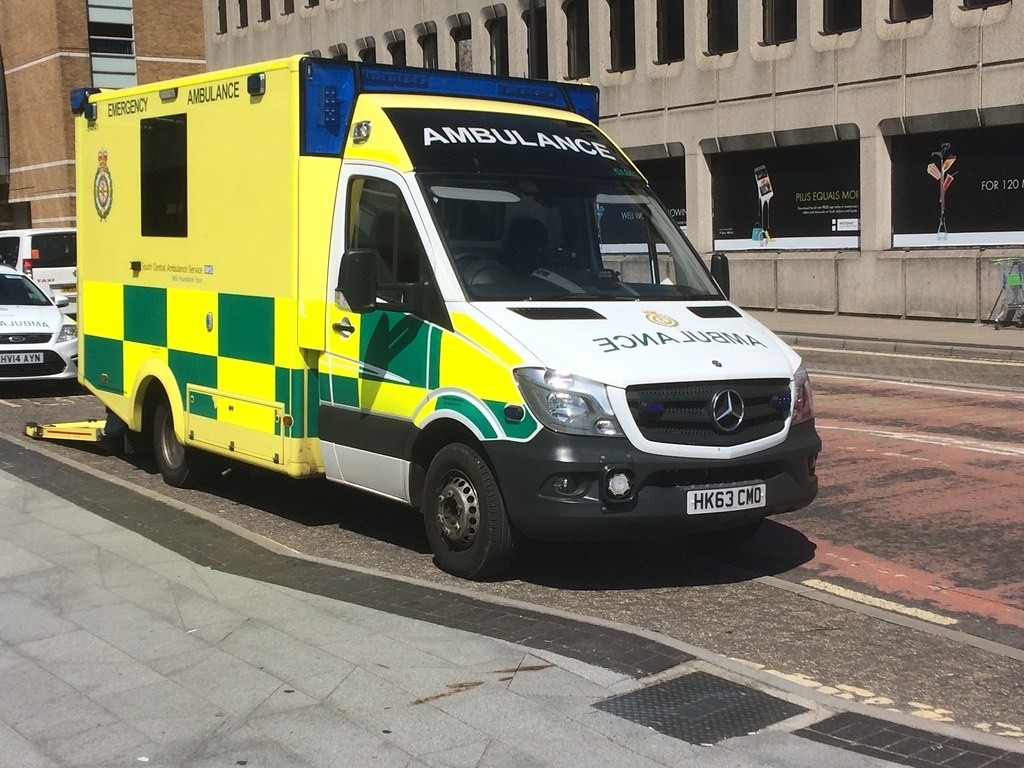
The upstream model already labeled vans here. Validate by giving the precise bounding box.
[1,226,79,316]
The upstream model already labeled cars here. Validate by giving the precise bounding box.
[1,266,81,384]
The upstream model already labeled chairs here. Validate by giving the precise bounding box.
[499,218,554,279]
[369,211,418,282]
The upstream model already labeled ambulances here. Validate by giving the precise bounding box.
[22,54,823,577]
